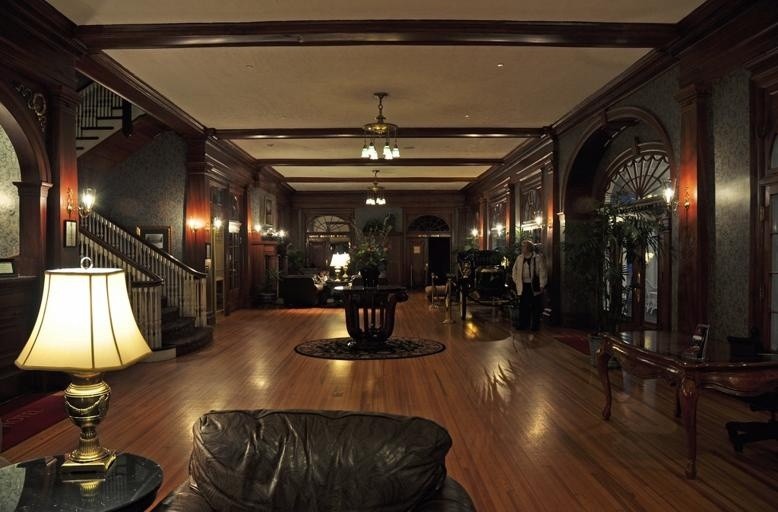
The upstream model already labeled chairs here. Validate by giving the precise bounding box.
[279,270,323,306]
[150,409,476,512]
[725,336,778,451]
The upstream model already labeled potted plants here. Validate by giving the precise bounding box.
[348,212,394,287]
[257,265,281,305]
[553,188,676,367]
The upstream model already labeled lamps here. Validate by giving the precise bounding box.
[364,171,386,206]
[663,180,690,214]
[330,252,345,282]
[14,256,153,474]
[67,185,96,220]
[358,93,403,160]
[341,253,351,278]
[188,217,208,263]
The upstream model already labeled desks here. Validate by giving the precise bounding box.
[330,286,409,346]
[594,329,778,479]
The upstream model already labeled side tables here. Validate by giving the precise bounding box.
[0,453,164,511]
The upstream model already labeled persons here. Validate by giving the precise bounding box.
[511,241,548,343]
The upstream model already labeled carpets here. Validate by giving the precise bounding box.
[295,337,446,361]
[555,337,591,355]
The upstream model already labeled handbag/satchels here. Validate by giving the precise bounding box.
[533,276,541,292]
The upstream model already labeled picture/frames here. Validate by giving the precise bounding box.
[135,223,173,255]
